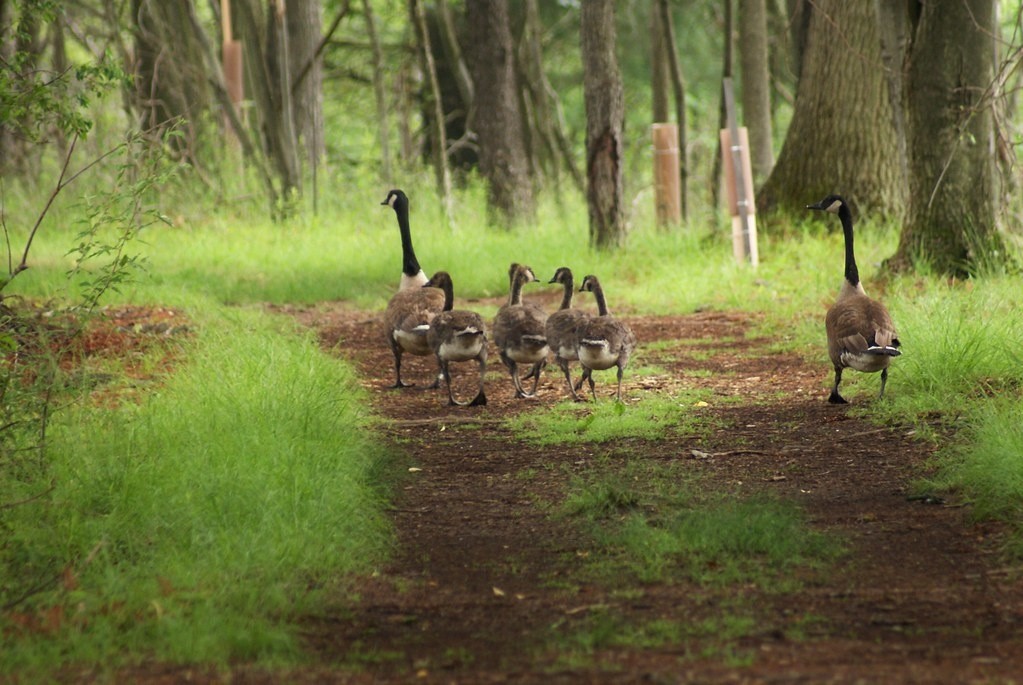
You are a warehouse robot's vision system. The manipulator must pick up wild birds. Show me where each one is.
[381,190,446,389]
[806,194,903,405]
[421,263,635,407]
